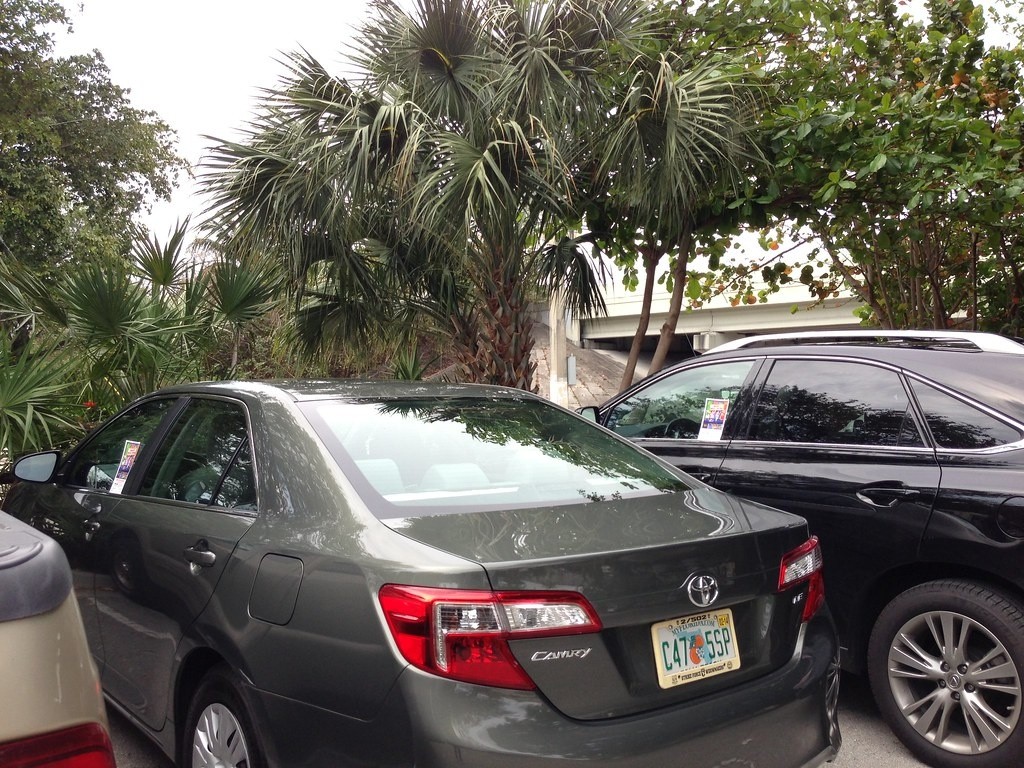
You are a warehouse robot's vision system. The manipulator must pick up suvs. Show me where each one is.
[570,331,1024,768]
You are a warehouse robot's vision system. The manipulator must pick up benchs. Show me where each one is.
[864,385,939,446]
[356,449,569,494]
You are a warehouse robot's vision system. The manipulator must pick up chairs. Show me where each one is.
[173,412,254,512]
[754,383,805,442]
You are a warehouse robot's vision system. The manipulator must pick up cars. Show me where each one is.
[1,379,844,768]
